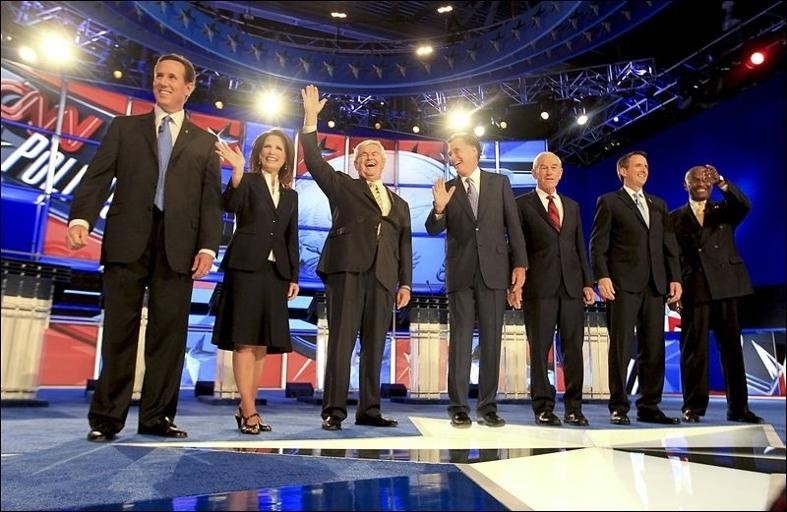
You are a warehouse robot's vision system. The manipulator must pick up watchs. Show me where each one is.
[716,174,726,188]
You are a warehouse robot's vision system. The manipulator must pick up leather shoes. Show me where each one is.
[532,410,561,427]
[477,412,507,428]
[87,429,116,442]
[449,411,473,430]
[319,415,342,431]
[609,411,631,426]
[636,409,702,425]
[355,416,399,427]
[136,425,189,438]
[564,413,590,426]
[725,402,768,424]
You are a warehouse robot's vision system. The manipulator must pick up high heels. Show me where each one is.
[232,400,271,436]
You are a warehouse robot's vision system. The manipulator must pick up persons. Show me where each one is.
[588,149,684,424]
[300,82,414,432]
[667,162,765,424]
[212,127,300,434]
[62,51,225,444]
[506,151,596,426]
[425,132,530,426]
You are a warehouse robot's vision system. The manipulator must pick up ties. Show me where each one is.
[149,114,179,214]
[694,201,706,227]
[464,179,481,227]
[365,178,385,243]
[633,193,650,230]
[545,195,561,234]
